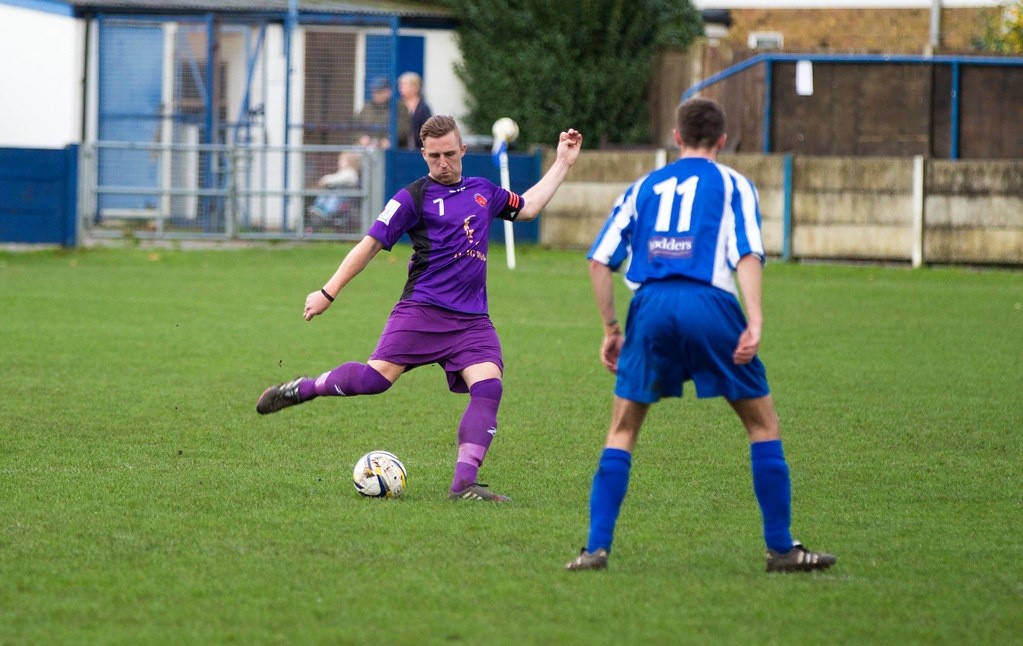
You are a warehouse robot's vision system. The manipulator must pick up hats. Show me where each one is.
[370,77,391,91]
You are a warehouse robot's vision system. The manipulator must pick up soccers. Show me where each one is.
[353,450,408,499]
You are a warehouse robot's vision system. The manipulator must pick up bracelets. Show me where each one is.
[320,289,336,302]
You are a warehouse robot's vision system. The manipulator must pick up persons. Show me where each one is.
[398,72,431,149]
[309,152,360,217]
[256,114,582,500]
[356,79,409,150]
[565,97,838,575]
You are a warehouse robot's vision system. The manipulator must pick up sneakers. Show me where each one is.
[564,546,608,572]
[256,375,309,414]
[447,482,511,501]
[765,543,836,573]
